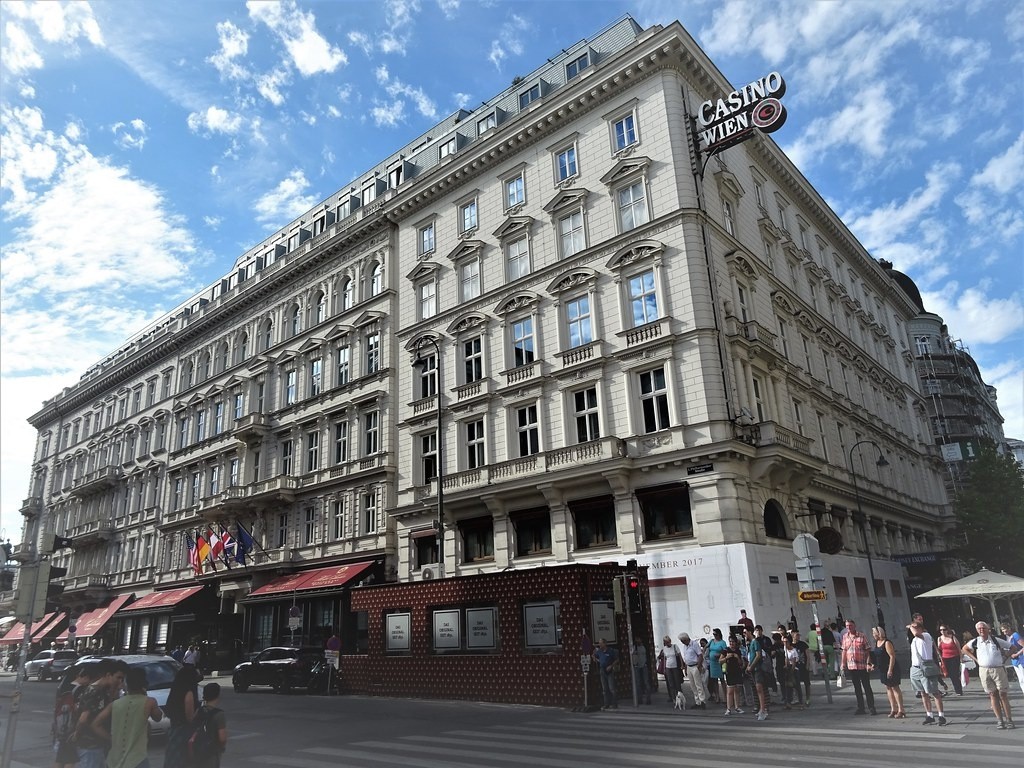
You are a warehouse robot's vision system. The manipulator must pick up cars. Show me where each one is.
[232,646,327,694]
[23,649,79,681]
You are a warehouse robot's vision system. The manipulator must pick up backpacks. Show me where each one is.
[184,702,223,762]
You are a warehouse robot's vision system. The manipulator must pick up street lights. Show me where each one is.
[849,440,892,639]
[416,337,446,579]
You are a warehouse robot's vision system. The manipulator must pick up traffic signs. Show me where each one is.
[798,589,826,602]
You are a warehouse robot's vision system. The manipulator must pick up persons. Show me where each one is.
[592,639,619,709]
[658,620,849,720]
[961,631,977,670]
[169,644,202,669]
[50,659,162,768]
[632,636,652,704]
[937,623,963,696]
[963,621,1017,729]
[906,613,944,698]
[999,622,1024,693]
[840,619,877,715]
[164,666,203,768]
[871,626,906,718]
[200,683,227,768]
[906,622,946,726]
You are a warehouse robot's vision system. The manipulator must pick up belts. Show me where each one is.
[688,664,696,667]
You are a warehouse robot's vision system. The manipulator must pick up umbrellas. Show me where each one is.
[914,566,1024,635]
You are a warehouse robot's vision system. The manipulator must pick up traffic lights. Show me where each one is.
[627,576,643,614]
[11,496,73,625]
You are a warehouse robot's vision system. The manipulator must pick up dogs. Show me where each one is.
[674,691,687,710]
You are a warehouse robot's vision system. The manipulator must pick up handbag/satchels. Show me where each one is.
[920,660,940,677]
[657,660,664,673]
[674,645,686,668]
[54,740,80,763]
[940,660,949,678]
[959,662,970,686]
[836,670,846,688]
[722,663,727,673]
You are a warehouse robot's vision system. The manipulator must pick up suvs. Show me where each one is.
[57,650,207,737]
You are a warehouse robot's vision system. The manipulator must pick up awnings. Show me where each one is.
[120,585,204,611]
[55,593,135,640]
[246,560,375,596]
[0,612,56,645]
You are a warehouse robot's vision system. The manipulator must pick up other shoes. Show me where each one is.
[609,704,617,709]
[870,707,876,715]
[755,710,768,720]
[1005,721,1015,729]
[997,721,1004,729]
[691,692,815,712]
[603,704,609,709]
[855,709,865,715]
[725,709,731,716]
[939,717,946,726]
[922,716,935,725]
[734,708,744,713]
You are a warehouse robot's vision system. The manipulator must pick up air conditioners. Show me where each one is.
[420,563,446,581]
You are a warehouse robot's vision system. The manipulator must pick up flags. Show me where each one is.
[221,528,236,562]
[235,525,253,564]
[186,534,199,571]
[207,528,224,560]
[196,531,210,562]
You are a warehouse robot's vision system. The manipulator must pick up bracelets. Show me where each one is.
[866,662,871,665]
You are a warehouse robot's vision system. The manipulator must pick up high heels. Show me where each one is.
[893,708,906,718]
[888,711,898,718]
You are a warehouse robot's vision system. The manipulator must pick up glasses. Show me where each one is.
[939,629,947,631]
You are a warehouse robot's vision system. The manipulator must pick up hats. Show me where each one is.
[203,683,220,700]
